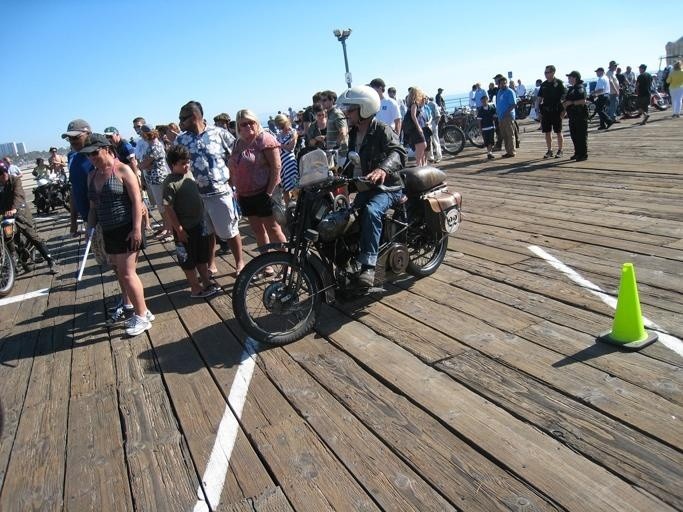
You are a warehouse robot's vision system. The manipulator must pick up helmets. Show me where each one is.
[0,160,8,174]
[338,86,380,119]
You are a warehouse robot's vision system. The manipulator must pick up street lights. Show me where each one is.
[334,27,353,88]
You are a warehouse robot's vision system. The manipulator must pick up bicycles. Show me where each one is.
[516,91,534,119]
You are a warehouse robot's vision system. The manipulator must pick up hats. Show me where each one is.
[567,71,581,78]
[80,133,111,153]
[103,127,118,135]
[141,124,156,132]
[595,68,604,72]
[610,61,619,65]
[62,120,91,139]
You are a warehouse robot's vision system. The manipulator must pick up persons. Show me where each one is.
[1,147,67,273]
[469,61,683,161]
[62,77,449,336]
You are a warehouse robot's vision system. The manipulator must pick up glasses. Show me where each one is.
[180,114,193,122]
[241,120,254,126]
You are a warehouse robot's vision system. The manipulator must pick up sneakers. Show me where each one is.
[571,154,587,161]
[544,150,554,158]
[487,153,494,159]
[358,265,375,287]
[598,119,619,129]
[502,154,515,158]
[555,150,563,158]
[107,298,154,336]
[48,261,59,273]
[640,115,649,124]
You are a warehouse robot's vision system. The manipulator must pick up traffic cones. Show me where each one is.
[596,263,661,354]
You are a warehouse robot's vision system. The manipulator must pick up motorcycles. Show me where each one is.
[436,105,487,155]
[0,204,39,296]
[586,73,671,122]
[231,146,462,347]
[32,167,72,214]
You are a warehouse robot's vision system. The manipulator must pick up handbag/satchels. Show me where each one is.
[403,110,420,133]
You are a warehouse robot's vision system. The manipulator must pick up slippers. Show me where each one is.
[190,282,221,298]
